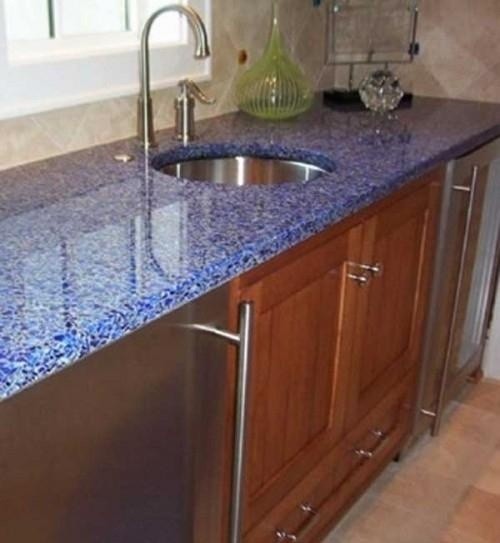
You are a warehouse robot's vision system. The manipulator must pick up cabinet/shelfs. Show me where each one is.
[224,164,447,540]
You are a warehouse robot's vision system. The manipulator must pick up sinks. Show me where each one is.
[147,137,338,189]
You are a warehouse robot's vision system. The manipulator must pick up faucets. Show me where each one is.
[134,2,210,147]
